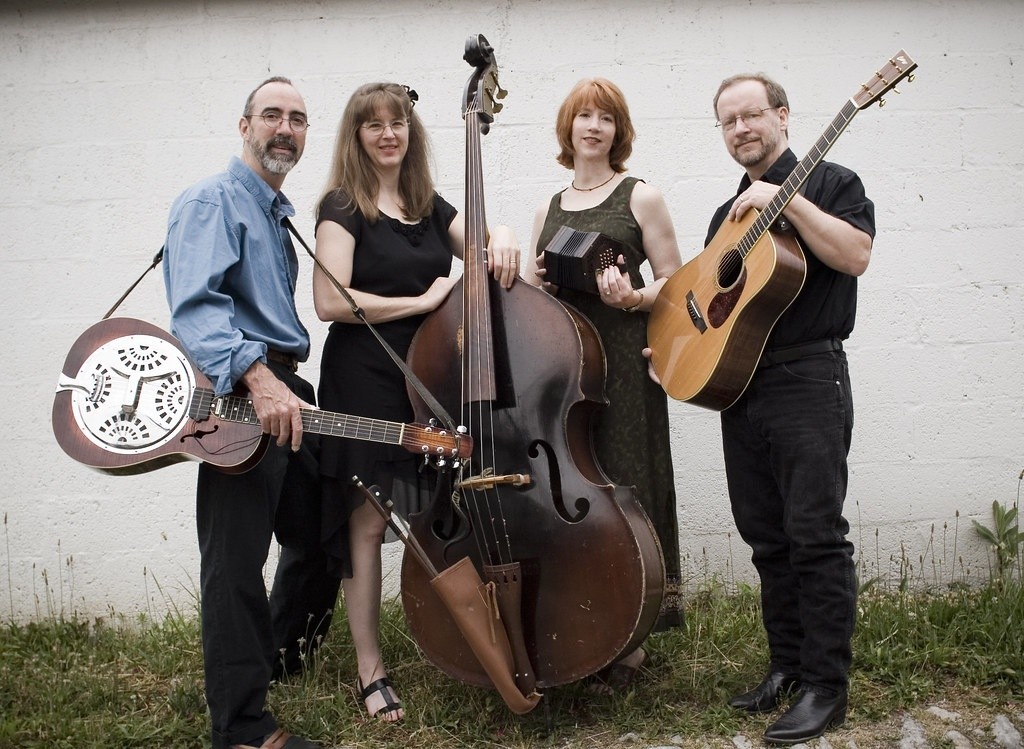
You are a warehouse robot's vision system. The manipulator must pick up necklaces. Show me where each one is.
[571,170,617,191]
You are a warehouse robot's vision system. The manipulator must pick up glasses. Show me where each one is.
[245,112,311,132]
[715,106,773,132]
[360,122,411,136]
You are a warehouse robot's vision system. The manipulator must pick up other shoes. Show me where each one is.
[226,728,322,749]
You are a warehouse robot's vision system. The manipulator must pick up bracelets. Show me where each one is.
[621,288,644,312]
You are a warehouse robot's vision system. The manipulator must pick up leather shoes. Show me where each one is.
[762,685,847,743]
[728,670,804,715]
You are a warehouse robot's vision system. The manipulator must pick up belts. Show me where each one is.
[263,350,298,372]
[757,339,843,366]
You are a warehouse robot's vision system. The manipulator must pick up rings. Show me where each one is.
[510,261,516,263]
[739,196,743,202]
[606,292,611,295]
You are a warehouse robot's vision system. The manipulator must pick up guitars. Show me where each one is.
[49,315,475,479]
[643,47,922,415]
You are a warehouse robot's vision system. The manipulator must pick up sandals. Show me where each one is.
[356,674,405,725]
[586,643,651,698]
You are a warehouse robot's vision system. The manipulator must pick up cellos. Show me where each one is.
[395,30,669,735]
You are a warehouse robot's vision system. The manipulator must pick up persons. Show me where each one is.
[522,80,683,698]
[646,72,876,742]
[313,82,519,722]
[163,77,332,749]
